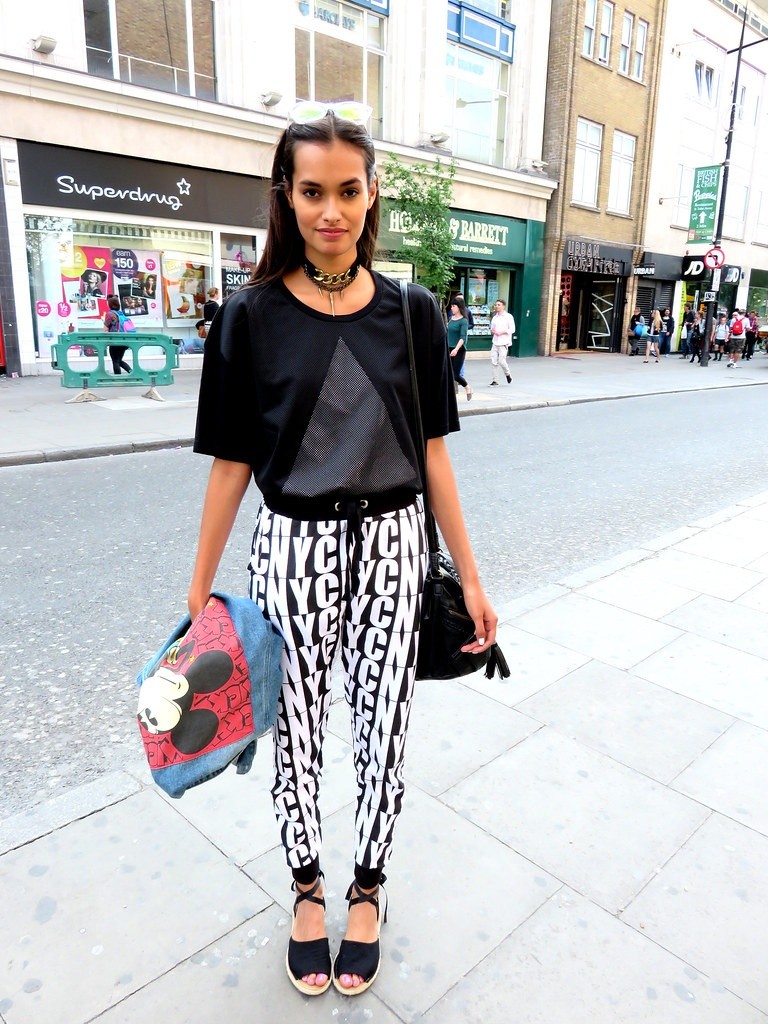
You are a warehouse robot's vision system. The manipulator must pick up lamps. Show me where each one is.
[260,90,283,108]
[525,156,549,173]
[427,132,451,147]
[31,34,58,53]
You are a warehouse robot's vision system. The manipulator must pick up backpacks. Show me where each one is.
[111,310,136,334]
[732,316,746,335]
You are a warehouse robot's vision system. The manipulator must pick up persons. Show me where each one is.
[628,307,645,356]
[487,299,515,388]
[81,270,107,297]
[680,303,758,369]
[447,298,472,402]
[652,307,674,357]
[643,309,663,363]
[103,295,132,375]
[188,101,497,996]
[204,287,219,336]
[143,275,155,298]
[449,293,474,376]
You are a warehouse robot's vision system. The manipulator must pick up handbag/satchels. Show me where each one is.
[634,323,649,338]
[691,325,703,349]
[414,551,510,684]
[681,324,687,338]
[198,325,206,338]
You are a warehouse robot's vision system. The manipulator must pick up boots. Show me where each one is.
[713,350,718,361]
[717,352,724,361]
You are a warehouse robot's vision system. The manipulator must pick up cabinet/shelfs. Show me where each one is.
[472,313,490,326]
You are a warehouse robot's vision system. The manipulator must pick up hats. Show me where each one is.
[739,309,746,313]
[82,268,108,283]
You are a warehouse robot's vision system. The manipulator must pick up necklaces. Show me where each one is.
[301,255,360,317]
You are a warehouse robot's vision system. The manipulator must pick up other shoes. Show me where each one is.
[507,374,511,383]
[467,387,473,401]
[629,347,711,364]
[490,382,499,388]
[727,361,734,367]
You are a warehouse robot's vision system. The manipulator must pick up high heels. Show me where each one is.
[286,874,335,996]
[330,881,391,994]
